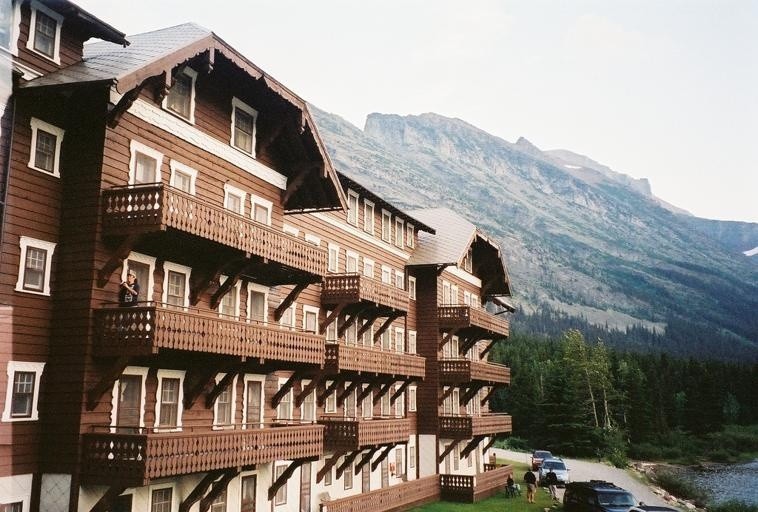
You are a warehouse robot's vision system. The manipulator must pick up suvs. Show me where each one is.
[627,504,680,512]
[561,477,641,512]
[530,449,555,470]
[538,456,569,486]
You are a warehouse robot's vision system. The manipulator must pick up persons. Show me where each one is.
[524,466,538,504]
[507,473,521,496]
[545,468,559,500]
[119,269,139,338]
[490,452,497,470]
